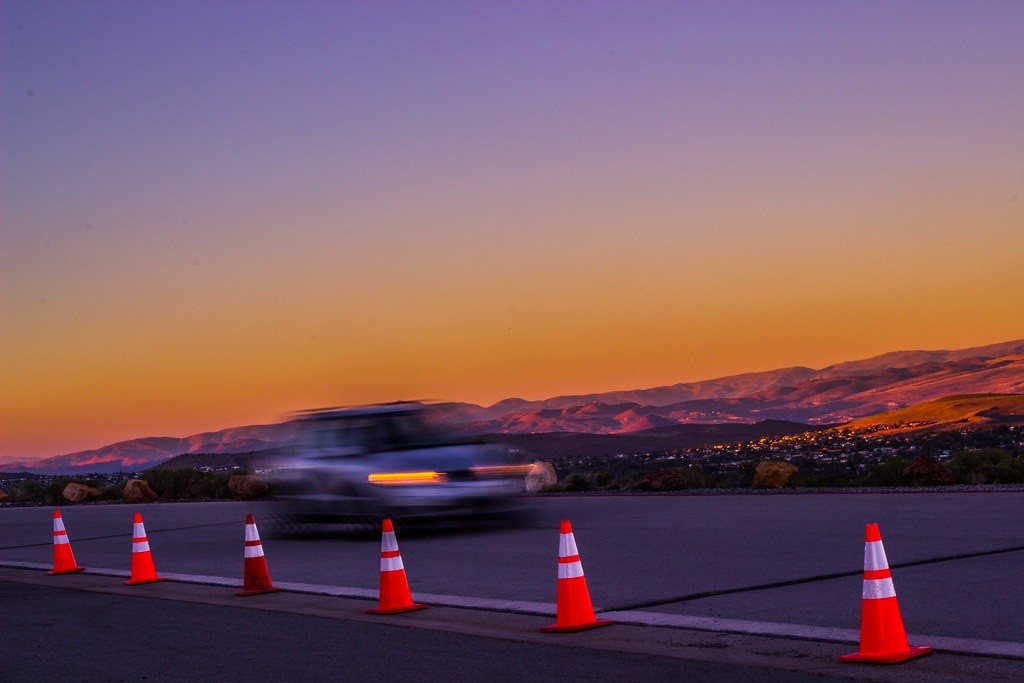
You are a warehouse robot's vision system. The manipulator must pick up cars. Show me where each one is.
[278,401,559,533]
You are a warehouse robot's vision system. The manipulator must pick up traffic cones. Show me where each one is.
[361,517,427,615]
[121,511,168,586]
[838,523,930,662]
[231,514,286,597]
[539,519,612,630]
[45,510,88,574]
